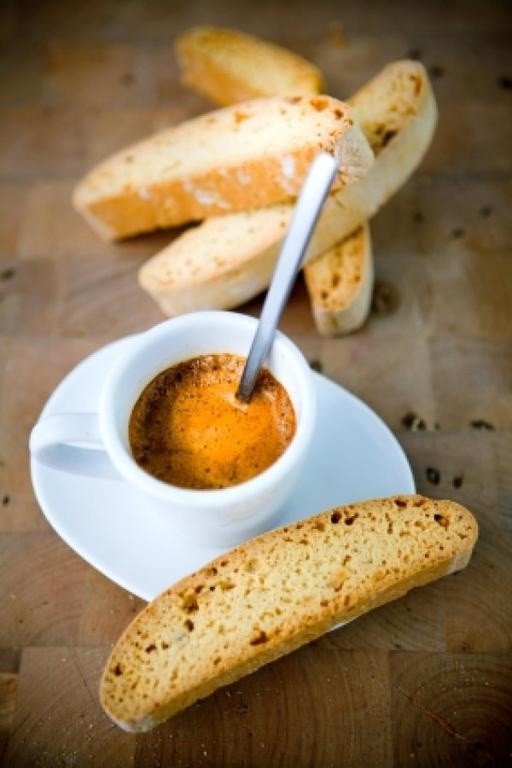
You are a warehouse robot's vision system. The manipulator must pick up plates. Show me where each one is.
[28,334,416,634]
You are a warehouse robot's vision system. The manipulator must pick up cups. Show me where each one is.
[31,308,320,548]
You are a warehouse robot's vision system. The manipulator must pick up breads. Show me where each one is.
[72,90,375,245]
[301,223,374,336]
[137,60,439,315]
[177,27,323,107]
[98,494,479,734]
